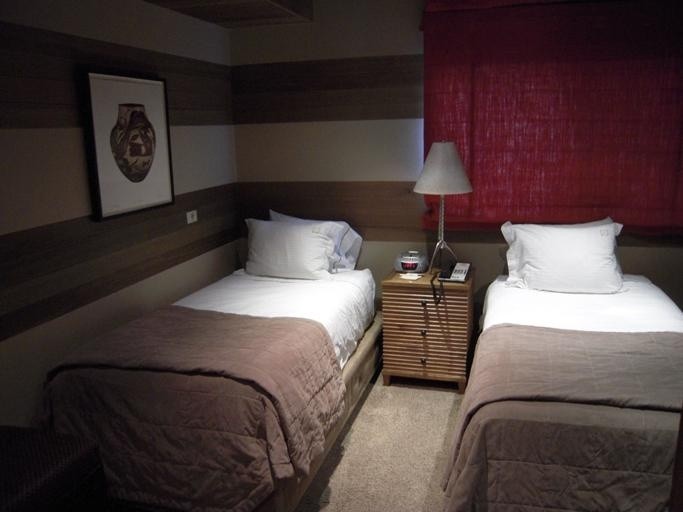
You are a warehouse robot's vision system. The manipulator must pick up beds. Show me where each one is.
[30,266,387,511]
[472,272,683,509]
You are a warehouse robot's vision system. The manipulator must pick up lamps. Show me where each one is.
[410,140,472,277]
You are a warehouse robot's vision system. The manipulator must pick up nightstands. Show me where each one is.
[377,264,476,398]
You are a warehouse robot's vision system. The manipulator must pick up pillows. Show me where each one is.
[500,220,627,296]
[556,213,612,228]
[266,208,362,276]
[240,216,351,285]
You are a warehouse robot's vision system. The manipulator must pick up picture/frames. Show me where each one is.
[83,67,177,223]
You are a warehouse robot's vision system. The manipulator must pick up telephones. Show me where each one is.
[438,259,472,282]
